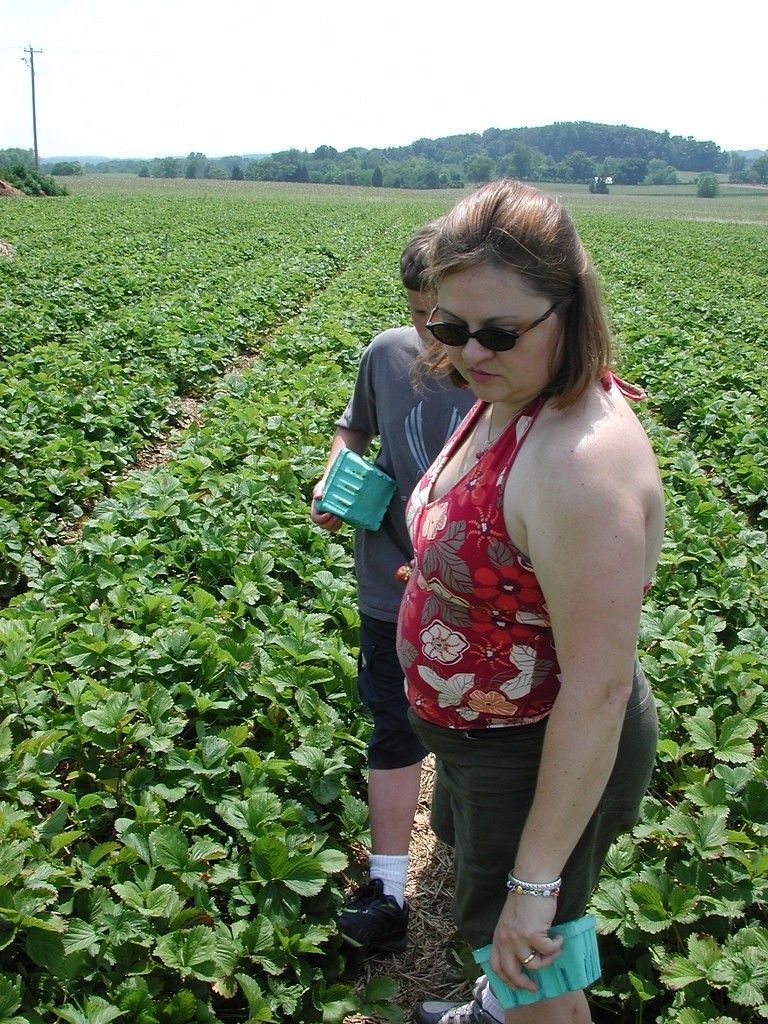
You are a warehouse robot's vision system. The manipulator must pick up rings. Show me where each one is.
[519,949,536,964]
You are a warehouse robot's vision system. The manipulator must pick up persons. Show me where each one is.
[395,181,664,1023]
[310,219,475,953]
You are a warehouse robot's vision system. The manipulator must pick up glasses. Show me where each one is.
[425,303,561,354]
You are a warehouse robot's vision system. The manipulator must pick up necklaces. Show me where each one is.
[475,399,530,462]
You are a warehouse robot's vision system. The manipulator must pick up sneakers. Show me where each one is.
[415,974,502,1022]
[334,877,412,953]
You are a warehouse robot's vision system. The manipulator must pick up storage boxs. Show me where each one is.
[314,447,398,532]
[471,914,603,1008]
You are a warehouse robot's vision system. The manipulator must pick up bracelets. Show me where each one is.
[506,871,562,898]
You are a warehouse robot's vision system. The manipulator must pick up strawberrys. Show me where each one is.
[395,564,411,582]
